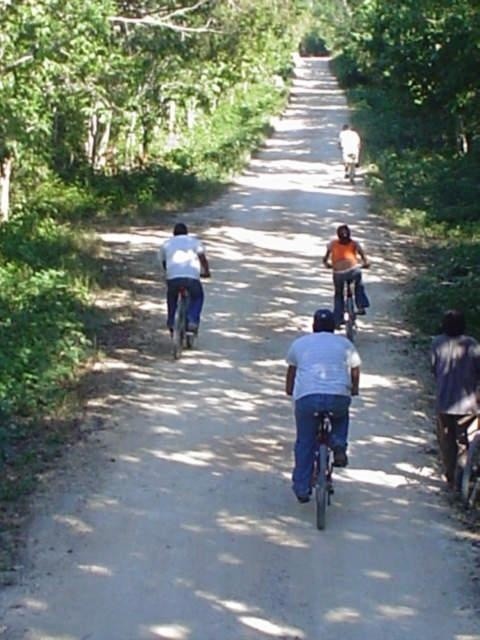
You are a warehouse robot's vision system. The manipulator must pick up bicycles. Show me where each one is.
[336,141,357,183]
[287,389,356,528]
[318,262,371,342]
[164,269,210,361]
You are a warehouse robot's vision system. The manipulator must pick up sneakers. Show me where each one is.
[334,447,346,466]
[360,306,364,312]
[297,486,312,503]
[187,325,196,333]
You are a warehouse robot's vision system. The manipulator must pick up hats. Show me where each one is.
[442,309,464,330]
[314,310,331,322]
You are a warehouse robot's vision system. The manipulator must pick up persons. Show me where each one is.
[321,225,371,328]
[427,309,480,494]
[337,124,361,179]
[157,222,211,339]
[285,309,364,503]
